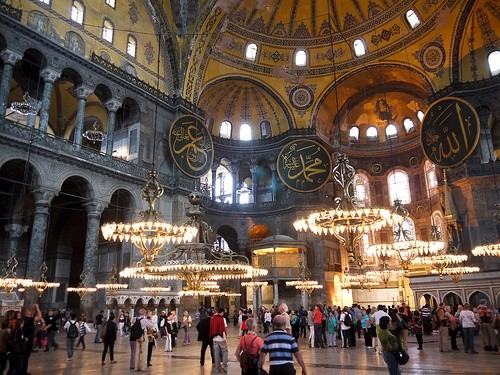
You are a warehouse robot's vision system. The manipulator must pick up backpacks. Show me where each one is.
[239,336,258,371]
[130,317,145,339]
[343,312,352,326]
[67,321,79,339]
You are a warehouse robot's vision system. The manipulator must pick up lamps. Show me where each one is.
[10,62,39,116]
[0,182,61,299]
[286,0,500,299]
[67,0,269,300]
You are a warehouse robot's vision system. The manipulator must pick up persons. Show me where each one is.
[101,314,117,365]
[378,316,401,375]
[0,299,500,375]
[209,308,229,373]
[235,318,265,375]
[257,315,307,375]
[63,313,79,361]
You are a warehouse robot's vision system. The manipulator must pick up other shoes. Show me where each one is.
[101,362,105,365]
[222,365,227,373]
[417,347,423,350]
[54,344,58,351]
[43,349,49,352]
[110,361,117,365]
[216,369,222,373]
[452,347,459,351]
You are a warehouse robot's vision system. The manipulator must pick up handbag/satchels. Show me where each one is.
[391,348,410,365]
[12,343,23,355]
[100,324,107,339]
